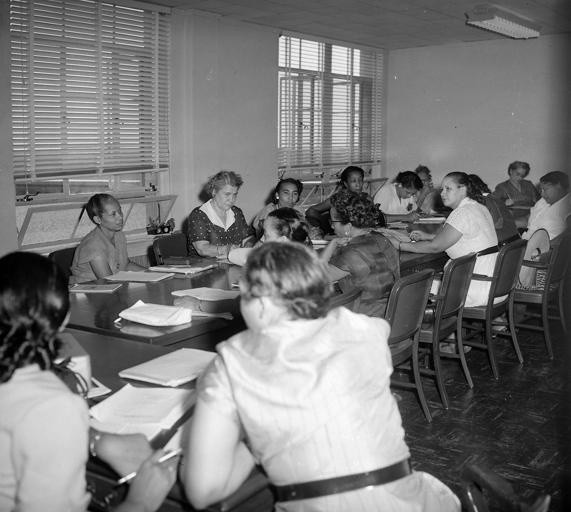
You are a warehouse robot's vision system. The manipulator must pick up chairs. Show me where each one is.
[330,285,362,316]
[388,268,435,424]
[47,246,77,268]
[152,234,188,267]
[418,251,478,411]
[515,233,570,361]
[463,239,526,381]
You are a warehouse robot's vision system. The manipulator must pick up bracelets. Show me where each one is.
[399,241,402,252]
[89,432,102,457]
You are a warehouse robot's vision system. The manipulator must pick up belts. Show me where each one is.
[273,459,411,503]
[499,234,519,246]
[477,245,499,257]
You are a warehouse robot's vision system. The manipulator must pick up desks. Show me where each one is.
[54,324,272,512]
[66,217,446,347]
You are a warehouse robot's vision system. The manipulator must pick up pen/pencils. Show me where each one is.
[506,192,511,199]
[117,447,181,485]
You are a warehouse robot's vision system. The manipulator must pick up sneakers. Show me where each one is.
[440,342,456,355]
[492,318,507,332]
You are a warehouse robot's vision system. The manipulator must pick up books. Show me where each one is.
[118,347,218,389]
[413,216,446,224]
[69,284,123,293]
[104,271,175,282]
[87,384,196,442]
[171,286,240,320]
[149,264,219,274]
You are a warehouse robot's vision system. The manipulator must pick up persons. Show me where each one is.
[319,189,401,318]
[386,172,509,354]
[186,171,256,259]
[373,171,426,223]
[0,251,180,512]
[473,174,521,334]
[494,161,540,206]
[180,240,463,512]
[69,194,147,286]
[259,206,315,250]
[521,172,570,240]
[414,164,443,216]
[306,166,375,235]
[252,178,307,238]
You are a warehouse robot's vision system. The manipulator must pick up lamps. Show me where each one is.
[464,7,542,39]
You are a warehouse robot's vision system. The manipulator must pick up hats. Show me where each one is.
[518,228,550,288]
